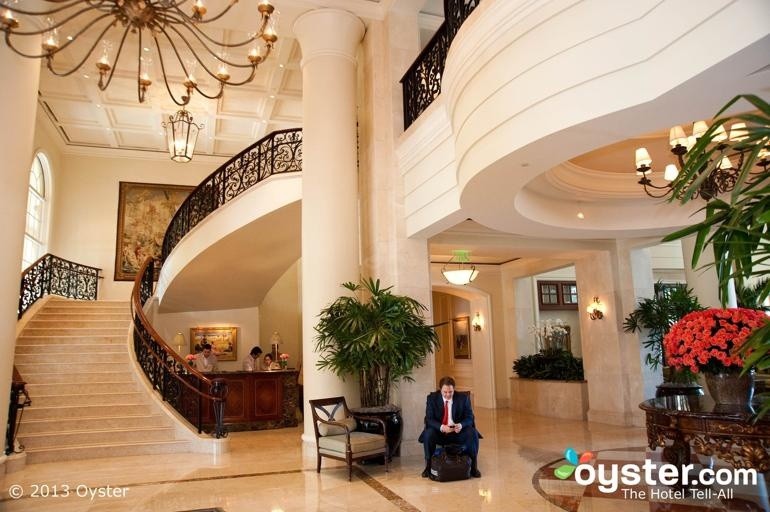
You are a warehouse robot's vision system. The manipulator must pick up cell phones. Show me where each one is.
[450,425,456,428]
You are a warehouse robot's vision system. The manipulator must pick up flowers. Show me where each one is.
[277,352,289,367]
[183,353,197,366]
[657,303,770,377]
[528,318,571,354]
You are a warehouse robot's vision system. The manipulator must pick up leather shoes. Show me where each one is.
[422,466,431,477]
[472,469,481,478]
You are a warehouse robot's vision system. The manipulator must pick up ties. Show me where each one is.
[442,401,448,426]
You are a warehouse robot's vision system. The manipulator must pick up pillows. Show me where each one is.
[318,418,359,436]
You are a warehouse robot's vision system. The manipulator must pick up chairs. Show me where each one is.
[308,394,391,482]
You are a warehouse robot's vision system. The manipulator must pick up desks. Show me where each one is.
[638,392,770,496]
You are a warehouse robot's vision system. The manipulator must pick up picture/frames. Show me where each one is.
[451,314,473,361]
[188,325,240,362]
[111,179,220,284]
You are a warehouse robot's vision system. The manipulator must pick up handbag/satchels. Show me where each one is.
[431,446,471,481]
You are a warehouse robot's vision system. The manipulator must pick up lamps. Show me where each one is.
[472,309,484,335]
[171,330,187,356]
[162,96,206,165]
[268,329,286,370]
[588,295,607,322]
[437,250,481,285]
[1,0,283,107]
[625,119,770,202]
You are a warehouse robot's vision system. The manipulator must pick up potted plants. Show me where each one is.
[621,276,710,398]
[312,275,442,465]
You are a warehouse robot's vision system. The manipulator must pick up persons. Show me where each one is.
[270,343,282,362]
[242,346,263,371]
[261,353,276,370]
[193,344,217,371]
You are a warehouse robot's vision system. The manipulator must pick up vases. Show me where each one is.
[699,366,754,415]
